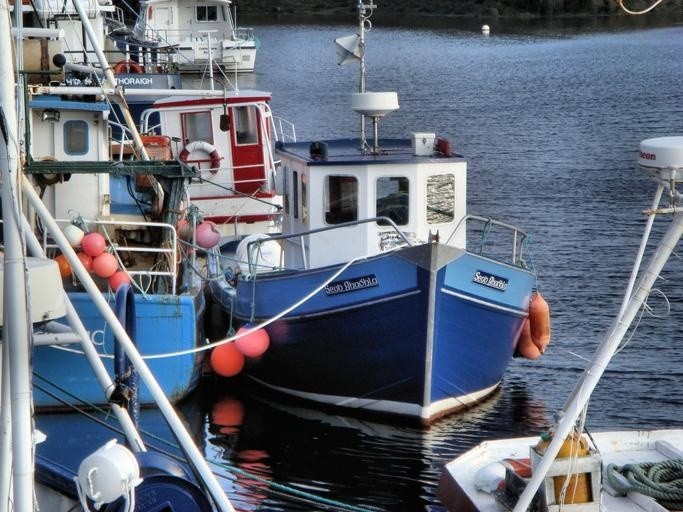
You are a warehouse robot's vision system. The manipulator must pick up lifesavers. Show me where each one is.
[181,142,220,183]
[114,59,144,74]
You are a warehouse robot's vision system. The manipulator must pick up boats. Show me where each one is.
[205,2,537,432]
[0,0,298,512]
[435,123,683,512]
